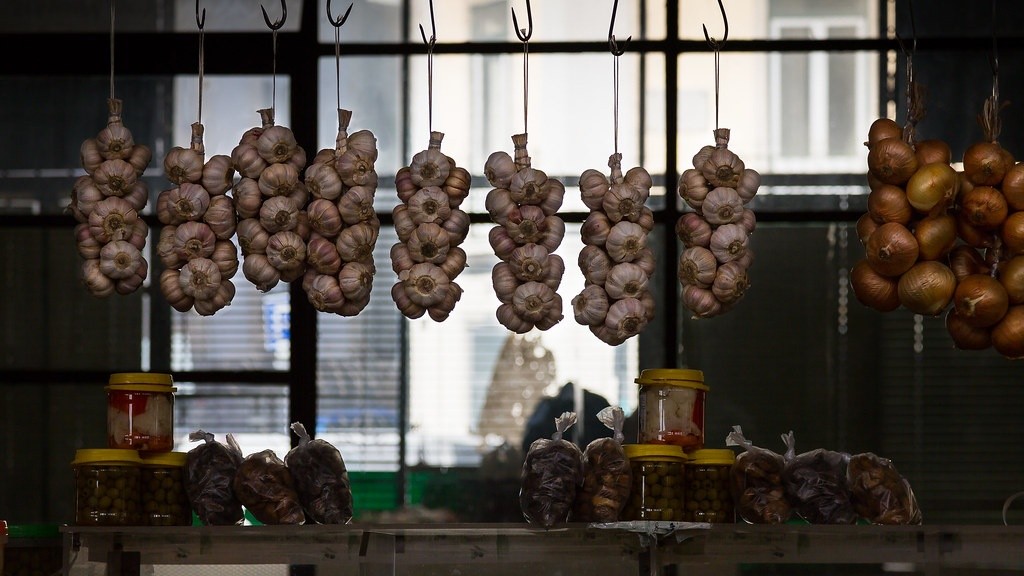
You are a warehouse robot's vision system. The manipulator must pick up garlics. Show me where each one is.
[75,100,759,345]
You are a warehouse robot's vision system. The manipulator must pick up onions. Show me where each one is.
[851,118,1024,358]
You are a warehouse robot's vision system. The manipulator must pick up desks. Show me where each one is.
[59,523,1024,576]
